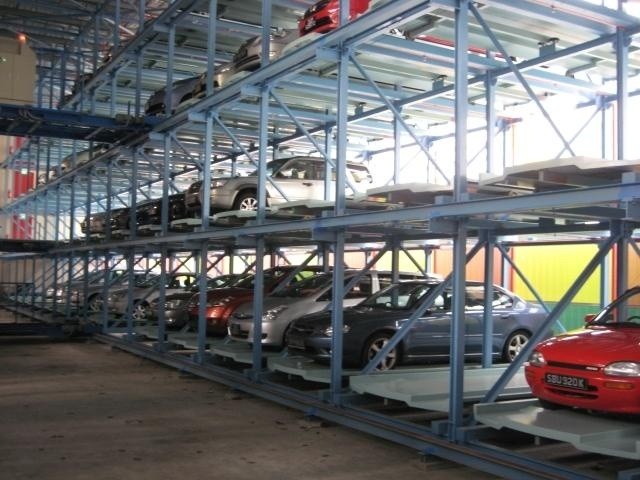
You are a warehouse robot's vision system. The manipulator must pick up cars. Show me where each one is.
[519,283,640,422]
